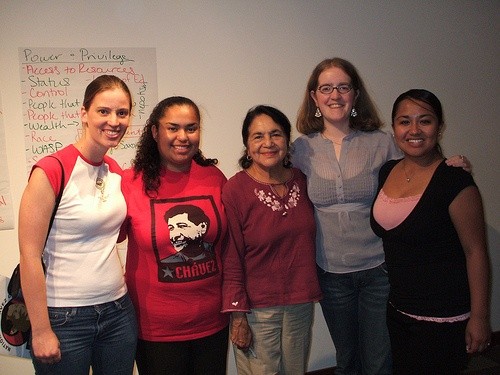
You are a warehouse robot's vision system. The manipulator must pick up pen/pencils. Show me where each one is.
[248,348,256,357]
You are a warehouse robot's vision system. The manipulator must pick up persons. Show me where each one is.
[19,75,139,375]
[289,58,473,375]
[220,105,323,375]
[369,89,492,375]
[116,97,230,375]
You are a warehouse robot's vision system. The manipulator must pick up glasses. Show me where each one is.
[315,84,355,95]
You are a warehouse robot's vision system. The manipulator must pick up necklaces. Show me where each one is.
[403,159,434,181]
[322,133,343,146]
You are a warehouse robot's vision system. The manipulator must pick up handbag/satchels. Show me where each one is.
[6,258,47,305]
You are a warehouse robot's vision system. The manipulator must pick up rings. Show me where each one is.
[487,342,490,345]
